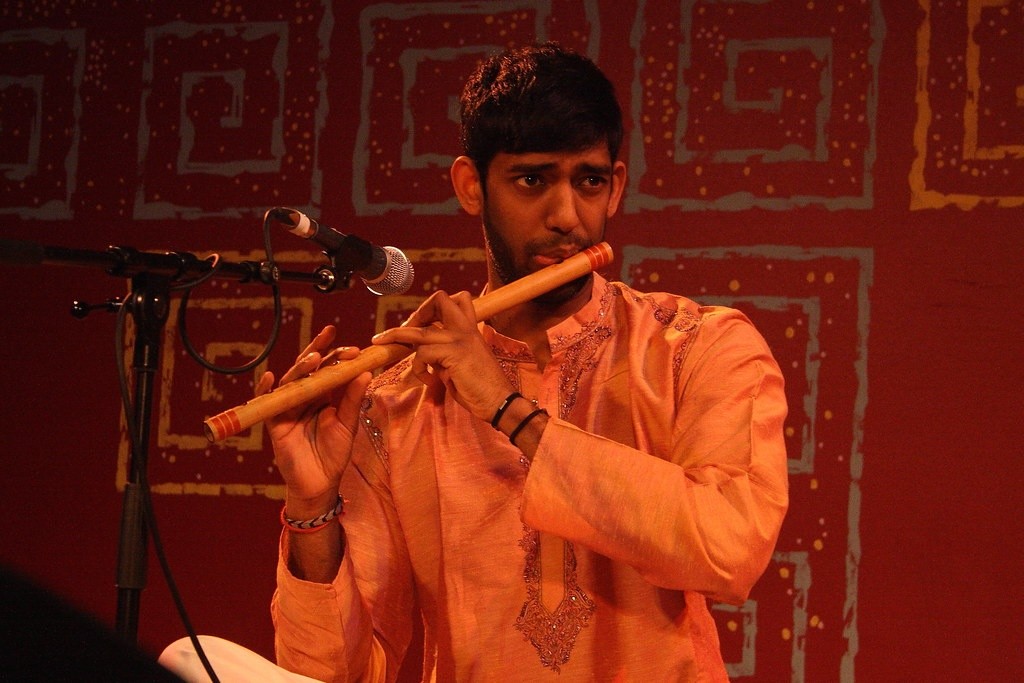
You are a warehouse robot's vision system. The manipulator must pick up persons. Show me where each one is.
[257,39,789,683]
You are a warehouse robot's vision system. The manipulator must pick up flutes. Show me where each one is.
[204,240,612,442]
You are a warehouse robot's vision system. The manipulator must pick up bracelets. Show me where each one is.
[508,409,549,446]
[278,496,355,535]
[489,391,522,429]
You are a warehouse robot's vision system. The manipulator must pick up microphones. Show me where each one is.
[273,206,415,298]
